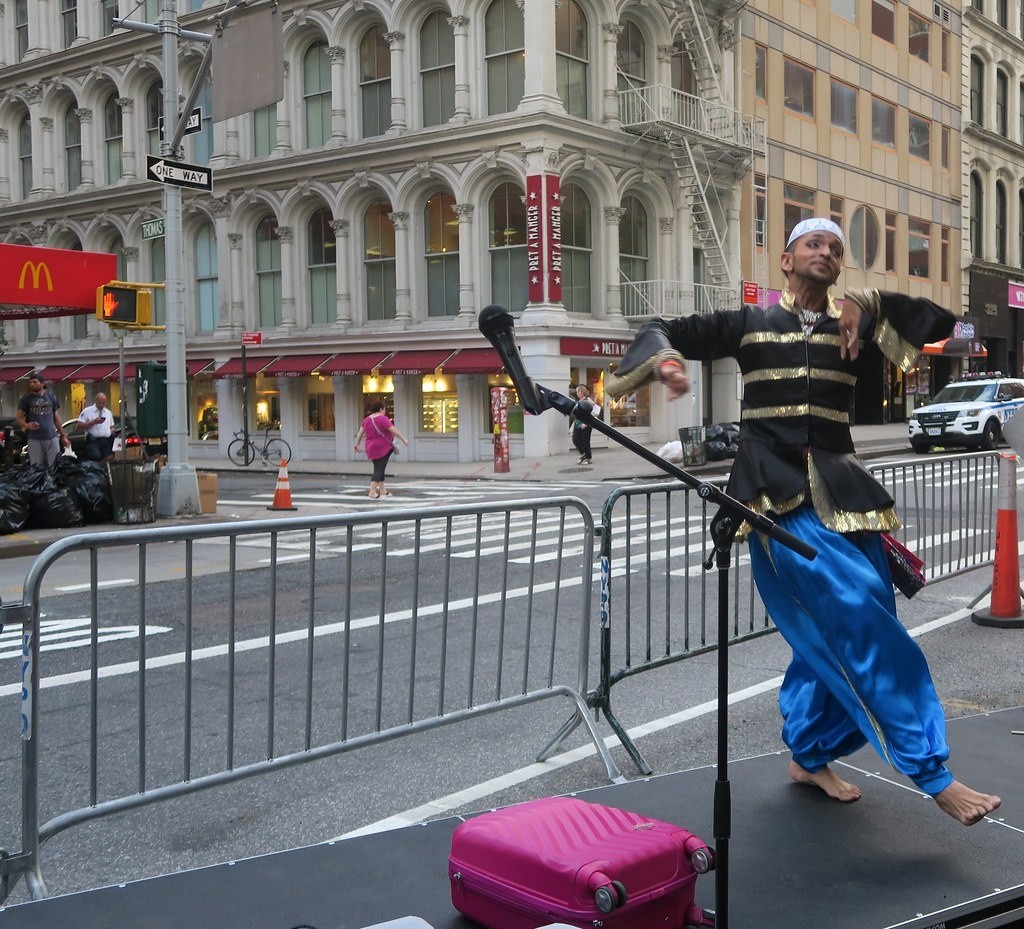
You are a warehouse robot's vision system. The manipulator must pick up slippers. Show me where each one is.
[367,495,383,501]
[379,491,392,496]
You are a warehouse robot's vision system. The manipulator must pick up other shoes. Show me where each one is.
[577,453,592,465]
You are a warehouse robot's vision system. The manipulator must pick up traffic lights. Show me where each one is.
[139,291,152,325]
[95,284,140,325]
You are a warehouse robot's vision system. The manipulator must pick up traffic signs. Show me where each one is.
[157,108,202,141]
[145,157,212,194]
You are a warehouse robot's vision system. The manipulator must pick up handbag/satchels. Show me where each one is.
[392,437,400,455]
[591,404,601,417]
[568,422,574,438]
[112,435,128,453]
[62,445,77,459]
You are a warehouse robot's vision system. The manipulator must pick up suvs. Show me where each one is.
[909,371,1024,454]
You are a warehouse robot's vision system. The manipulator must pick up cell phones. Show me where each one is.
[101,417,107,420]
[33,422,39,428]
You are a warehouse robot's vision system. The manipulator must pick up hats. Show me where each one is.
[784,218,845,261]
[30,374,47,389]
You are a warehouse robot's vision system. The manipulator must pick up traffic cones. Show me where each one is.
[266,458,298,511]
[971,453,1023,629]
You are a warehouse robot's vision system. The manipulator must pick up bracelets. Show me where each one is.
[26,423,29,430]
[60,433,67,437]
[657,359,686,380]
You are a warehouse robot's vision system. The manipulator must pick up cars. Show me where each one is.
[0,416,27,464]
[21,418,166,462]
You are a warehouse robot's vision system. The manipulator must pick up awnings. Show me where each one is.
[211,348,506,380]
[920,335,987,358]
[0,358,215,382]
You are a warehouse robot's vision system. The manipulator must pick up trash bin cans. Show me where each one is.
[108,460,159,524]
[679,427,708,467]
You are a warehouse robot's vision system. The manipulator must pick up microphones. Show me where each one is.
[478,304,544,415]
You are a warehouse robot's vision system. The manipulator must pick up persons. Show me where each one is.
[75,392,116,462]
[15,374,72,470]
[354,401,408,498]
[571,385,595,466]
[602,213,1005,827]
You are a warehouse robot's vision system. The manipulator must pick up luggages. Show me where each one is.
[448,797,716,929]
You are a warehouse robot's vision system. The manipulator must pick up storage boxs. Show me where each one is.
[195,471,217,512]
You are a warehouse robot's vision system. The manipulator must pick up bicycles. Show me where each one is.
[227,426,292,466]
